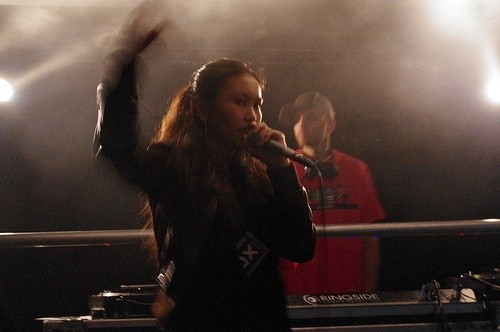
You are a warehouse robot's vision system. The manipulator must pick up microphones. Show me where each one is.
[245,127,317,168]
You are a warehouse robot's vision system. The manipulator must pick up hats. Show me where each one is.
[279,92,334,127]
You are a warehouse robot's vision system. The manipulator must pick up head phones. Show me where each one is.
[305,151,338,178]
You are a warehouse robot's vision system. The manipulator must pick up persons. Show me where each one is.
[92,7,318,332]
[272,91,387,297]
